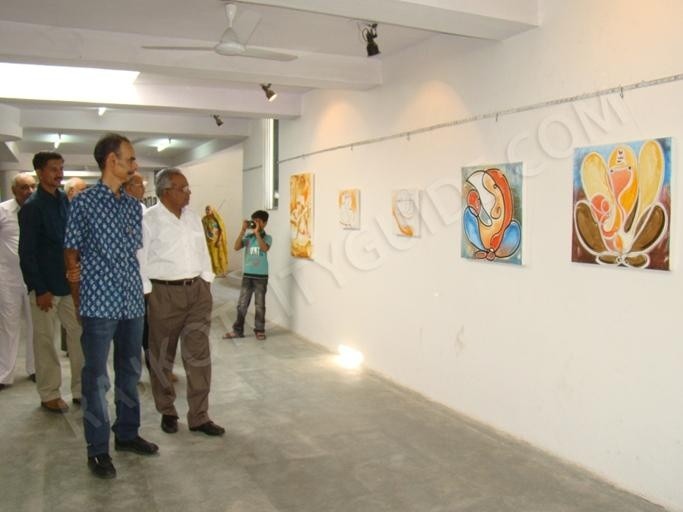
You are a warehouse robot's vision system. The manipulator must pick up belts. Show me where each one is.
[150,276,201,288]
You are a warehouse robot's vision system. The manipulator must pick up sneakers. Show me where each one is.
[88,453,116,479]
[114,434,161,455]
[0,350,82,405]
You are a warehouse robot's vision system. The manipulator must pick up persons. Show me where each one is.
[123,172,178,382]
[64,177,88,201]
[18,152,84,413]
[201,205,228,276]
[0,173,39,389]
[221,211,272,340]
[65,134,158,477]
[137,168,226,436]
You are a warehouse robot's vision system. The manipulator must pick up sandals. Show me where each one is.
[222,331,245,339]
[255,330,266,341]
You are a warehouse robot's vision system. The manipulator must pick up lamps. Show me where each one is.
[362,23,381,57]
[213,114,225,129]
[260,83,277,102]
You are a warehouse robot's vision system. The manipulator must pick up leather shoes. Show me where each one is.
[189,420,226,437]
[41,398,69,413]
[160,413,179,434]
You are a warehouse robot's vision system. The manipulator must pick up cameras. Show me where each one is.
[246,221,255,228]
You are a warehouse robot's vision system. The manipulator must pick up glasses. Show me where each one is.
[164,185,189,194]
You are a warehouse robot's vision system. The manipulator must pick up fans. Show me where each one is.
[141,4,299,62]
